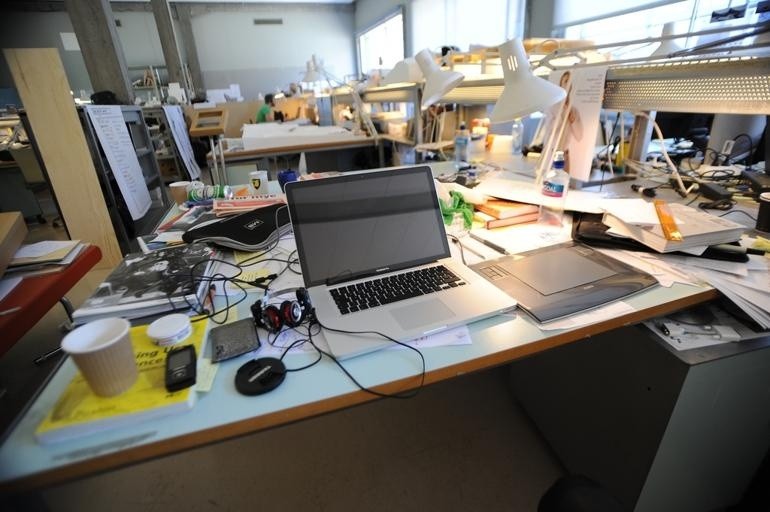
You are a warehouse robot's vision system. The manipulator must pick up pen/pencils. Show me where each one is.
[202,284,216,315]
[468,231,510,255]
[34,347,62,364]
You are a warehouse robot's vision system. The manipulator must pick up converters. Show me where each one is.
[699,182,733,203]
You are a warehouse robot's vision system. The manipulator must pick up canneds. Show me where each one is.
[188,185,233,201]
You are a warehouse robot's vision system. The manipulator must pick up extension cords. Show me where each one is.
[697,164,741,177]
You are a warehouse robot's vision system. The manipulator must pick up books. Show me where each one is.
[34,314,210,447]
[71,241,223,328]
[602,203,747,254]
[474,200,539,229]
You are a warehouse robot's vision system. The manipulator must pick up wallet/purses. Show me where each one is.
[209,317,261,364]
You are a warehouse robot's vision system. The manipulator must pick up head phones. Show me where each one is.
[251,286,308,333]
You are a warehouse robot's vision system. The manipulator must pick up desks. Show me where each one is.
[0,211,103,370]
[203,127,388,181]
[0,163,770,512]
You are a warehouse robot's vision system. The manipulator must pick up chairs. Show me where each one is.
[0,151,48,229]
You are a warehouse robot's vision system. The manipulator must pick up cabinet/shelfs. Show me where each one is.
[128,64,173,90]
[76,105,170,255]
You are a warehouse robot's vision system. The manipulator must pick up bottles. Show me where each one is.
[454,122,470,171]
[536,152,569,240]
[512,117,524,154]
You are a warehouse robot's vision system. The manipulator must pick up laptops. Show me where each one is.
[283,164,517,360]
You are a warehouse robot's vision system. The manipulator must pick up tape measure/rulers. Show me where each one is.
[653,199,683,241]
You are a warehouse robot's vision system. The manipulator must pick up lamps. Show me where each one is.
[301,54,364,96]
[486,35,582,127]
[415,49,468,114]
[273,84,304,99]
[648,21,688,56]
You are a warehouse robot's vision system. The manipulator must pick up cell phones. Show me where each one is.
[165,344,195,392]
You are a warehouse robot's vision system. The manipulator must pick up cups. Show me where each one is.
[754,192,770,234]
[276,170,297,192]
[249,170,269,196]
[169,181,190,207]
[61,317,139,400]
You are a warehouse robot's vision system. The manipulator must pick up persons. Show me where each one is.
[255,94,277,123]
[544,72,583,173]
